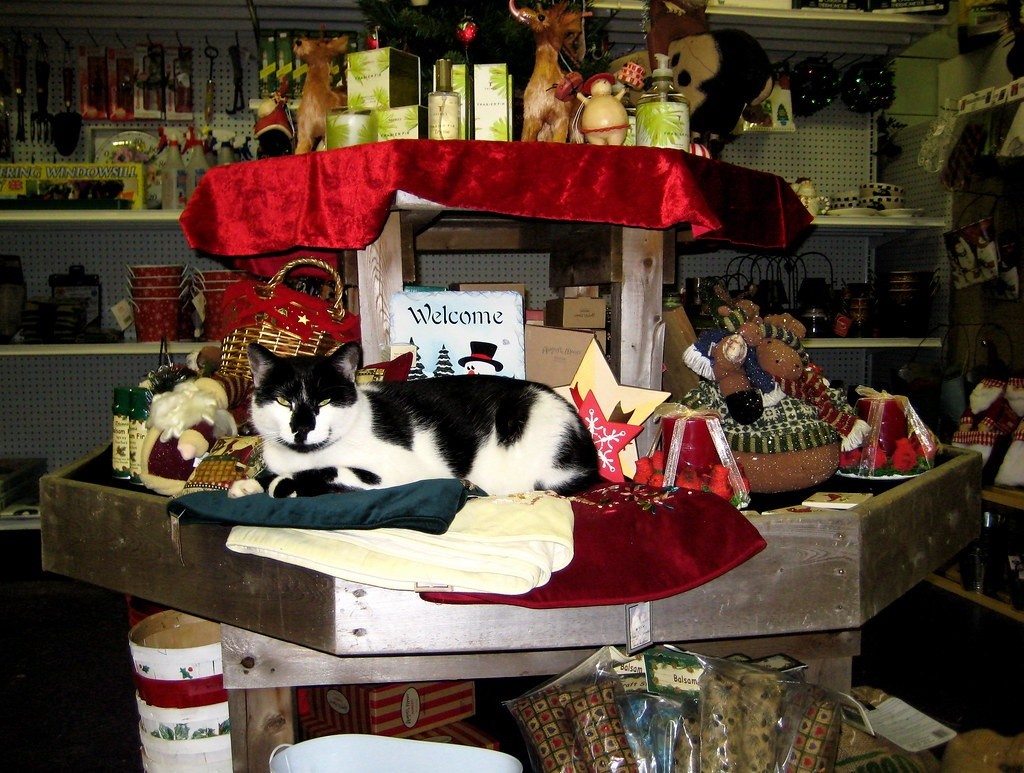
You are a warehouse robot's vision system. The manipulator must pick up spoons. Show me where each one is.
[53,38,82,157]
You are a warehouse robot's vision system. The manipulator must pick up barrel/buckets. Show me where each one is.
[128,609,235,773]
[265,734,523,773]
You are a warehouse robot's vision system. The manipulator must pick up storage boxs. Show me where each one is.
[123,260,248,344]
[296,680,500,756]
[326,47,515,145]
[451,278,608,392]
[216,260,363,383]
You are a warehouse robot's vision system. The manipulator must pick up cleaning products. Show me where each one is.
[635,50,691,153]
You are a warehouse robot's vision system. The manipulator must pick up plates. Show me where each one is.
[95,131,162,207]
[827,208,877,217]
[877,209,925,217]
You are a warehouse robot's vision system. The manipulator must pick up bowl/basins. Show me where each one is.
[830,201,860,209]
[861,183,906,197]
[859,195,906,208]
[831,190,859,201]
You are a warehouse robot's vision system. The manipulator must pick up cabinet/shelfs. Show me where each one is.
[38,138,982,773]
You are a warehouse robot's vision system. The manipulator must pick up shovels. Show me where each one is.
[53,68,82,157]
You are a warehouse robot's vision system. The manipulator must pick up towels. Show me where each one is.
[224,493,572,594]
[163,478,487,539]
[420,480,770,611]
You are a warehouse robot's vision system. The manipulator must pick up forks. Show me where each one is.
[30,42,53,147]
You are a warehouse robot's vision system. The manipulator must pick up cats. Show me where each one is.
[227,343,600,498]
